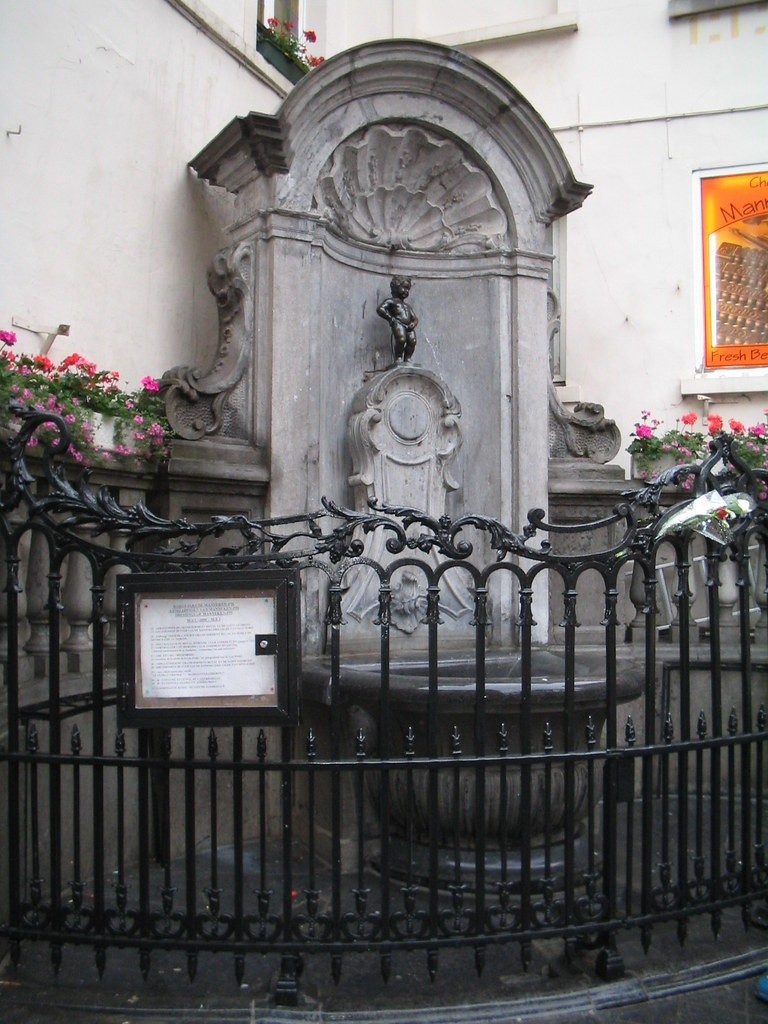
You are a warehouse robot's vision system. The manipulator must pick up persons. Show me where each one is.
[376,275,421,367]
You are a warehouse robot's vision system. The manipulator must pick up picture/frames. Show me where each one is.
[693,163,768,376]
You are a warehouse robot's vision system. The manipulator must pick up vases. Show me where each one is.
[0,410,168,472]
[629,451,709,481]
[255,39,309,85]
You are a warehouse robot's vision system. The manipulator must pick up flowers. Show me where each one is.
[625,409,768,500]
[0,329,176,465]
[266,17,329,67]
[614,490,759,558]
